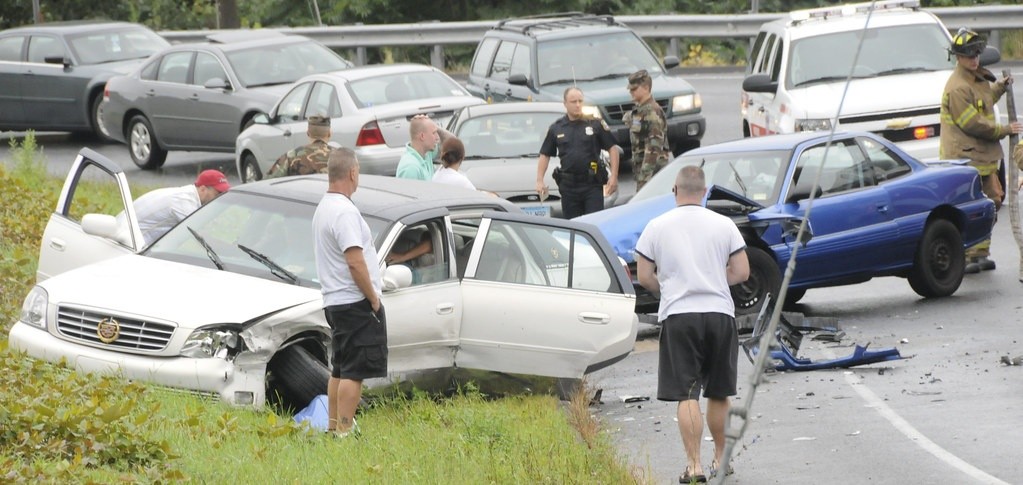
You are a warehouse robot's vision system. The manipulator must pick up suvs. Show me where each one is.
[468,16,707,154]
[741,0,1006,206]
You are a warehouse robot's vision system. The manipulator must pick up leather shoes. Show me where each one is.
[963,257,996,275]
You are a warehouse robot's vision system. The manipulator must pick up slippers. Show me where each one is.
[678,465,707,483]
[710,463,735,476]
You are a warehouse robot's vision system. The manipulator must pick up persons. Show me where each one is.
[384,230,432,265]
[396,115,477,190]
[114,169,231,250]
[536,87,620,220]
[635,167,750,483]
[312,148,389,445]
[622,69,675,191]
[265,114,336,178]
[940,28,1023,274]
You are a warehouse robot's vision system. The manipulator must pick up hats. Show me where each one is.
[440,136,465,159]
[307,112,331,126]
[194,169,230,192]
[626,70,648,89]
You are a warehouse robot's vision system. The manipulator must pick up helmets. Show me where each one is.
[948,28,987,56]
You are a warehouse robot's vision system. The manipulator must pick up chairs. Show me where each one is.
[385,82,410,102]
[165,64,188,82]
[794,166,828,194]
[392,238,421,285]
[467,133,499,156]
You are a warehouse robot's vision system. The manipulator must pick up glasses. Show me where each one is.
[952,41,986,57]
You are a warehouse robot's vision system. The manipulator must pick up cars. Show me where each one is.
[0,20,176,143]
[235,62,489,184]
[554,127,997,321]
[446,104,616,220]
[9,146,639,409]
[102,31,358,168]
